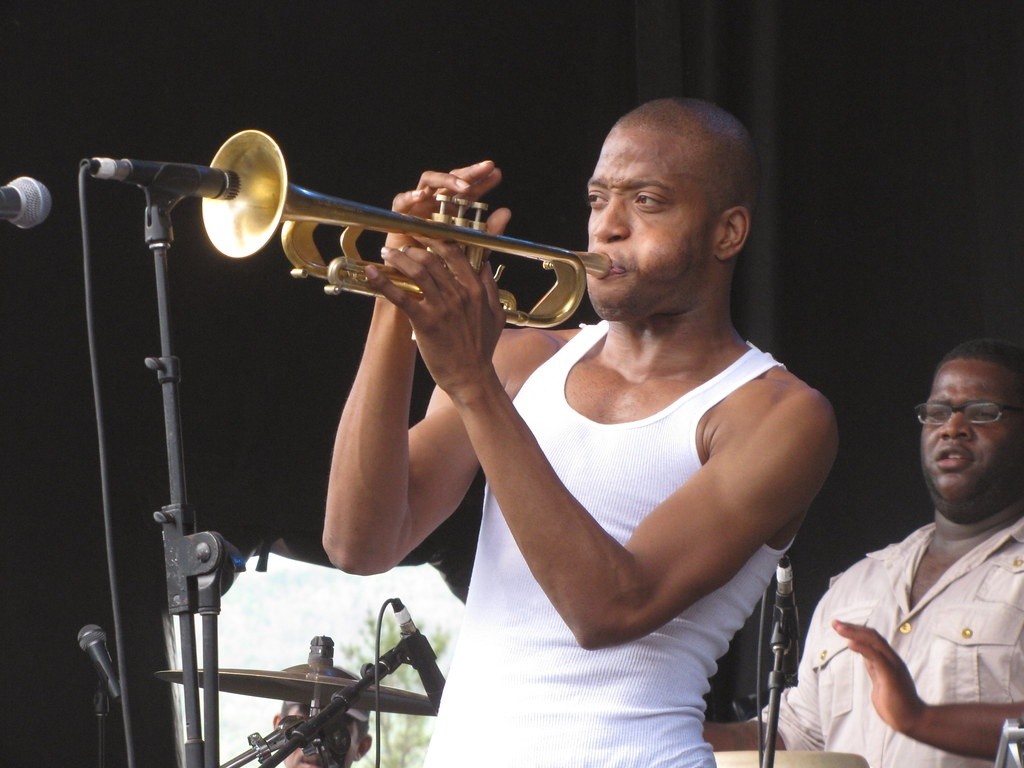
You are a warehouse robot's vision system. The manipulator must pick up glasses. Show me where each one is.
[912,402,1024,425]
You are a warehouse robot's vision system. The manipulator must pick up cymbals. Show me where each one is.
[151,664,439,717]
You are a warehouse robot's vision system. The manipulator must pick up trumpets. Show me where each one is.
[200,130,612,333]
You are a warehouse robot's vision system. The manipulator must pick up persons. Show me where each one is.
[322,100,838,768]
[704,339,1024,768]
[273,698,372,768]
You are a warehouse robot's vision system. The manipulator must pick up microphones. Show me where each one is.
[0,174,54,232]
[389,596,446,716]
[775,554,802,688]
[74,621,122,703]
[82,154,243,201]
